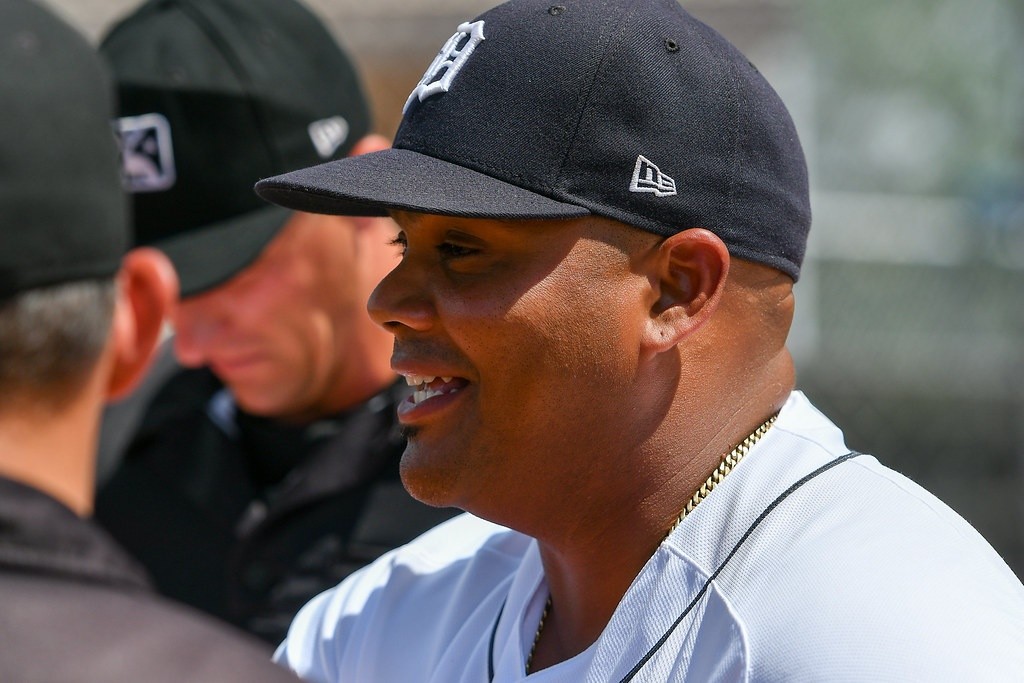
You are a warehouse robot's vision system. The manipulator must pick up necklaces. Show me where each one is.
[524,415,778,671]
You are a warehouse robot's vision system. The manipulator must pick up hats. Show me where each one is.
[0,0,127,296]
[96,0,372,299]
[254,0,812,282]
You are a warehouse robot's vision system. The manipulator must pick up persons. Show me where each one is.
[255,0,1024,683]
[0,0,302,683]
[92,0,467,664]
